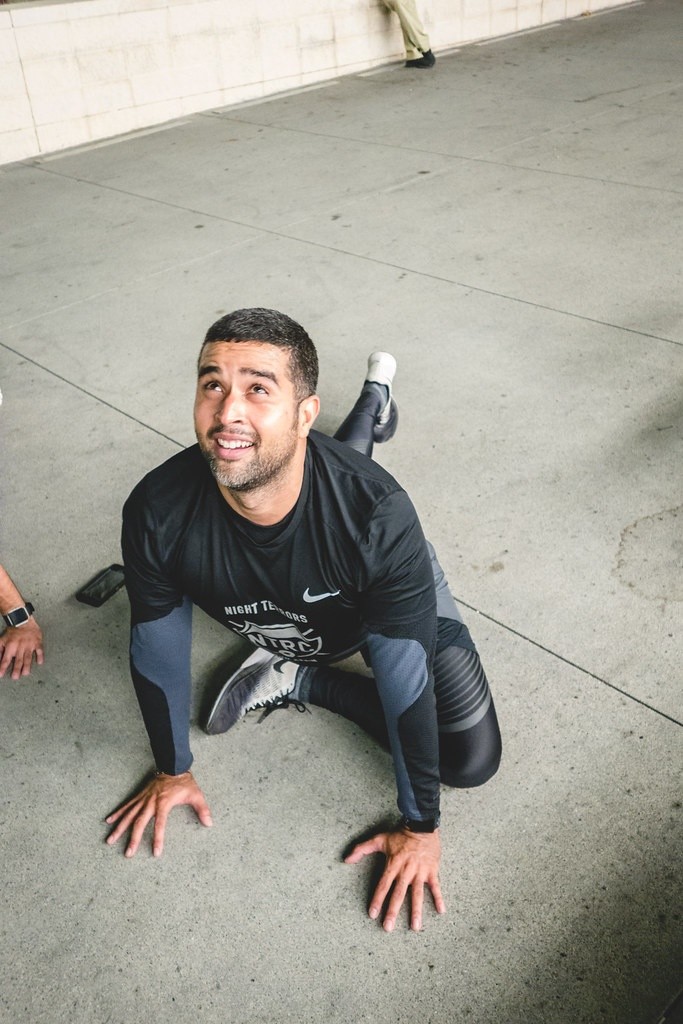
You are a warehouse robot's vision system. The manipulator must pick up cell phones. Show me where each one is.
[76,564,127,607]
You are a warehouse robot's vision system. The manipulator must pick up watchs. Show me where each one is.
[401,814,442,833]
[2,601,35,628]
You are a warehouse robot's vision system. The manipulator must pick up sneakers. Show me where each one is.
[205,647,309,737]
[365,352,398,444]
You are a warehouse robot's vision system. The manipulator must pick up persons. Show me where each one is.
[102,306,503,936]
[1,562,46,679]
